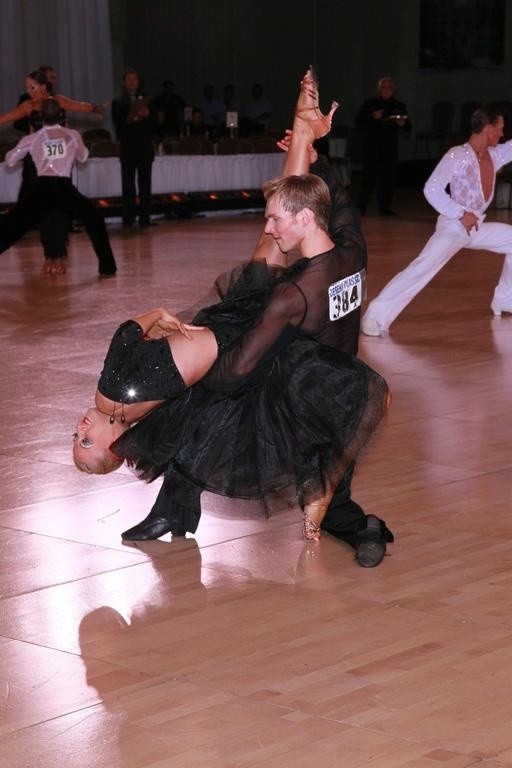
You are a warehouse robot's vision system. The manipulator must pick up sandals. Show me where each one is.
[41,260,66,275]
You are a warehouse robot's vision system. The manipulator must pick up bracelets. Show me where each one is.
[91,104,103,112]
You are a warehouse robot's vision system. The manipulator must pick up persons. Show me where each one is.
[75,64,389,549]
[351,75,412,213]
[1,102,117,279]
[110,66,160,227]
[154,80,272,136]
[14,63,59,137]
[358,103,511,338]
[1,70,106,273]
[119,94,393,571]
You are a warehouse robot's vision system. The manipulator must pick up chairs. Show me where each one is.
[0,119,287,161]
[415,100,483,153]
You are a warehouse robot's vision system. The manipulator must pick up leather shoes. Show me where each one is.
[139,219,160,226]
[122,214,135,225]
[359,204,367,216]
[98,267,119,275]
[379,207,397,218]
[360,315,380,337]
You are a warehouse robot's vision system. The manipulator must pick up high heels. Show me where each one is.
[356,509,387,567]
[295,64,340,141]
[120,509,188,541]
[490,298,512,317]
[287,477,329,544]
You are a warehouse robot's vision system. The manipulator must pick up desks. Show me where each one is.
[1,152,288,220]
